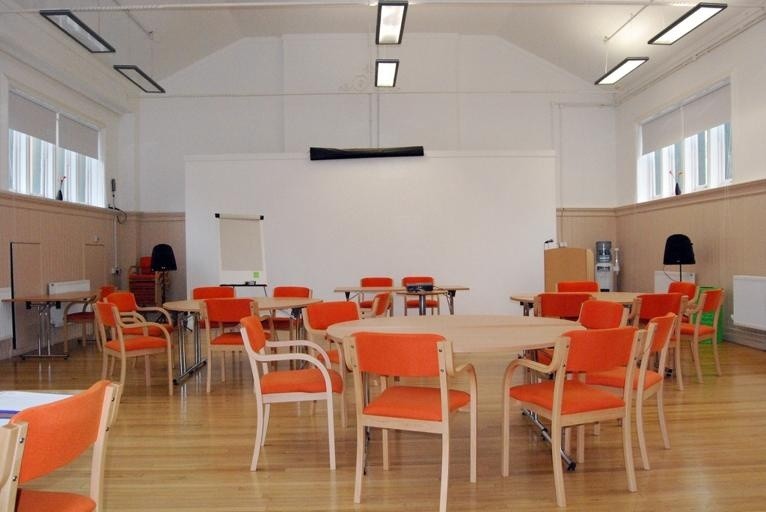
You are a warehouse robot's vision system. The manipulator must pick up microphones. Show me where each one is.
[544,239,554,243]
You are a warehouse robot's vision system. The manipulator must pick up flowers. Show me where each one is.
[55,174,66,191]
[669,168,685,183]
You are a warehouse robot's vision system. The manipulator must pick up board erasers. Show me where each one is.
[245,281,256,286]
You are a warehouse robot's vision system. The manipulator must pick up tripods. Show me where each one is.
[155,271,176,325]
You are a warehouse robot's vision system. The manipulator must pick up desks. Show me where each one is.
[1,292,97,359]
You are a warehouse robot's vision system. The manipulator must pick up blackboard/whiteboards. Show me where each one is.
[215,213,268,287]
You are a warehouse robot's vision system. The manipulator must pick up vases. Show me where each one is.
[676,182,682,195]
[54,190,63,201]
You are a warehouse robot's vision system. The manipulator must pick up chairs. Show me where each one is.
[92,302,174,398]
[2,377,125,512]
[125,256,168,307]
[102,291,177,371]
[163,255,726,511]
[62,282,118,358]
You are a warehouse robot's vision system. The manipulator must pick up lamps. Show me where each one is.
[149,244,178,325]
[594,17,650,86]
[375,58,402,89]
[645,1,729,48]
[374,1,411,46]
[663,232,698,281]
[111,18,170,96]
[37,2,117,55]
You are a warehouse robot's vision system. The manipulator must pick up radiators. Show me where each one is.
[730,273,766,332]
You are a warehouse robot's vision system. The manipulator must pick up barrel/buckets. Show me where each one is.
[595,240,613,264]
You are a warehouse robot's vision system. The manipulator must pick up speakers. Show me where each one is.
[663,234,695,264]
[150,244,177,271]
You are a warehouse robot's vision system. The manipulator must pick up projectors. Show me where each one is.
[407,283,434,292]
